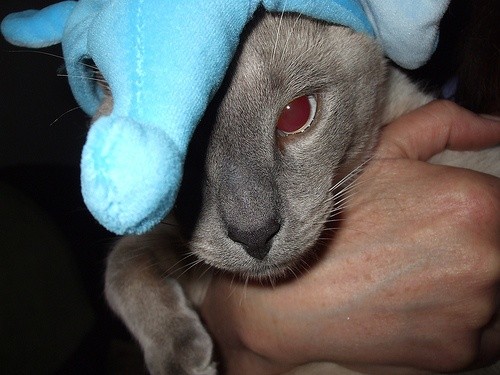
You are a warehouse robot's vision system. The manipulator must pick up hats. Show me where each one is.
[2,0,450,237]
